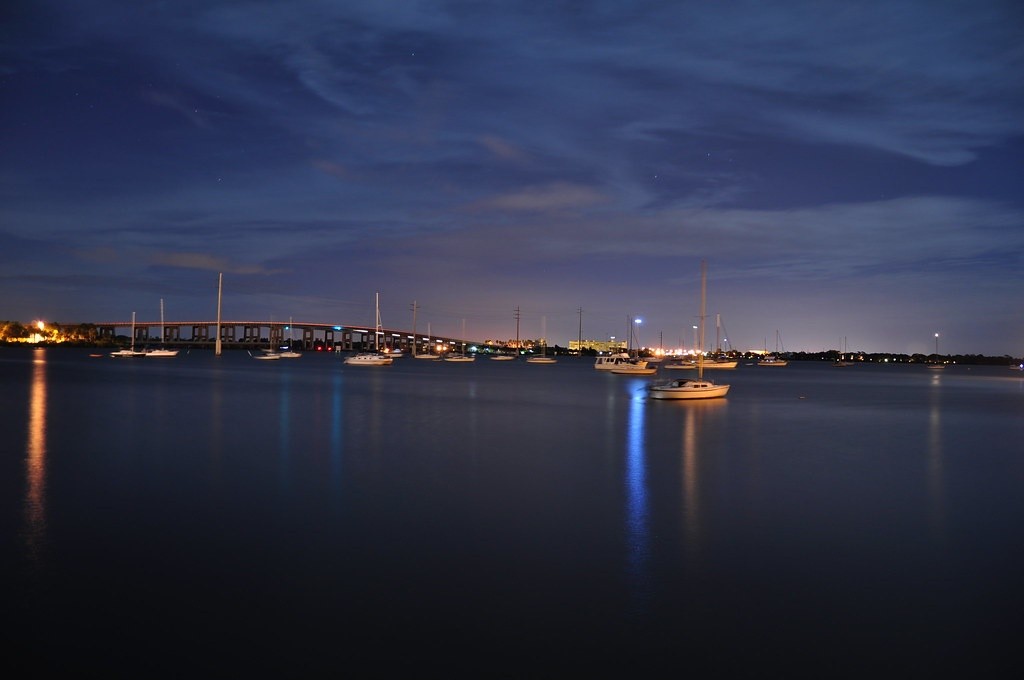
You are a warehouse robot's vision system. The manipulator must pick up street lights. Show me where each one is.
[935,333,938,358]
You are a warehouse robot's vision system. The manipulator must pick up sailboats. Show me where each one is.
[522,315,558,363]
[694,313,737,367]
[253,319,281,360]
[445,319,475,362]
[594,315,648,369]
[267,316,301,357]
[757,329,788,366]
[140,298,180,356]
[345,292,394,365]
[714,314,729,362]
[664,339,697,369]
[832,337,846,366]
[840,336,855,365]
[611,317,658,374]
[415,322,440,358]
[111,311,146,357]
[649,260,731,398]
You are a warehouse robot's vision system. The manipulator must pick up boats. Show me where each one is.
[384,353,404,357]
[88,353,103,357]
[1009,364,1024,369]
[491,356,514,360]
[644,357,662,361]
[927,365,946,369]
